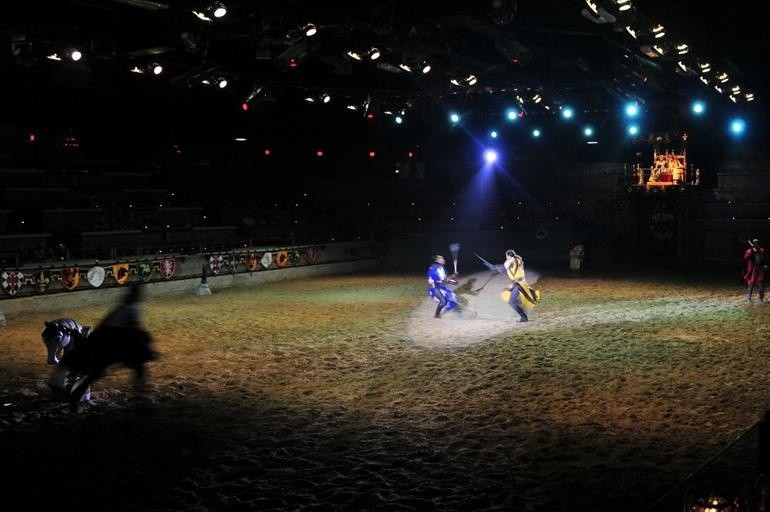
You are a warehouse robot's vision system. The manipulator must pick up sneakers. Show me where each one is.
[747,300,767,305]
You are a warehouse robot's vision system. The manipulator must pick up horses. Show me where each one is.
[41,318,96,404]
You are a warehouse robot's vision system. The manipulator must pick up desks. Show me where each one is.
[651,166,687,183]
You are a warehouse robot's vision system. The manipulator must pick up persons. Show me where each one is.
[742,238,767,302]
[490,249,541,322]
[66,282,159,413]
[426,254,461,318]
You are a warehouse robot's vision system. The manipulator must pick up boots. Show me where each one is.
[515,306,530,323]
[435,304,443,319]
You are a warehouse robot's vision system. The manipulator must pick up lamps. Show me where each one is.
[581,0,755,105]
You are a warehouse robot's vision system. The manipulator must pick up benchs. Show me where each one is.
[653,151,686,168]
